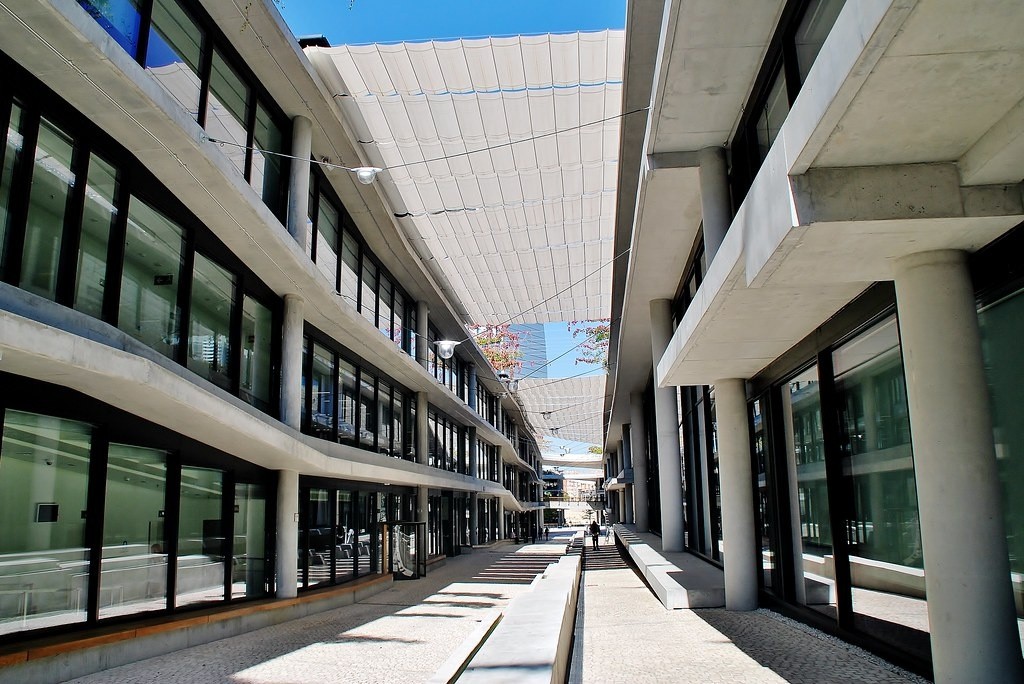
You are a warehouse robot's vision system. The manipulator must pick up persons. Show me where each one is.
[538,526,543,540]
[531,523,537,544]
[511,529,517,538]
[545,527,549,541]
[590,521,600,551]
[569,521,572,528]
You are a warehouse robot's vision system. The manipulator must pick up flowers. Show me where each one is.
[465,319,608,379]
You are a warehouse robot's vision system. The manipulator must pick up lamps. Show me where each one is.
[498,373,509,382]
[432,340,461,359]
[541,408,552,420]
[550,426,560,435]
[350,166,383,184]
